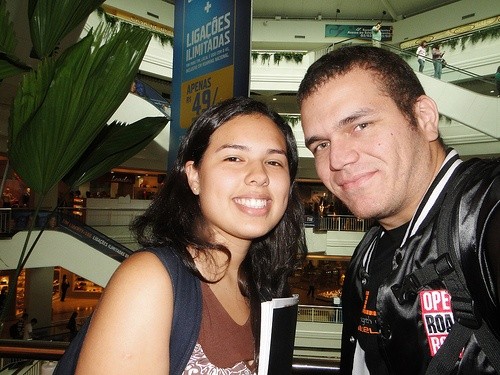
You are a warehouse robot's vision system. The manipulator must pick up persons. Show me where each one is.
[372,22,383,48]
[0,273,79,340]
[73,96,308,375]
[416,40,446,80]
[297,45,500,375]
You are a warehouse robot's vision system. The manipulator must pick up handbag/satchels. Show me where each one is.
[441,59,447,68]
[66,323,76,330]
[52,245,203,375]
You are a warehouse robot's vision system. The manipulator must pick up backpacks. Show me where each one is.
[9,321,23,338]
